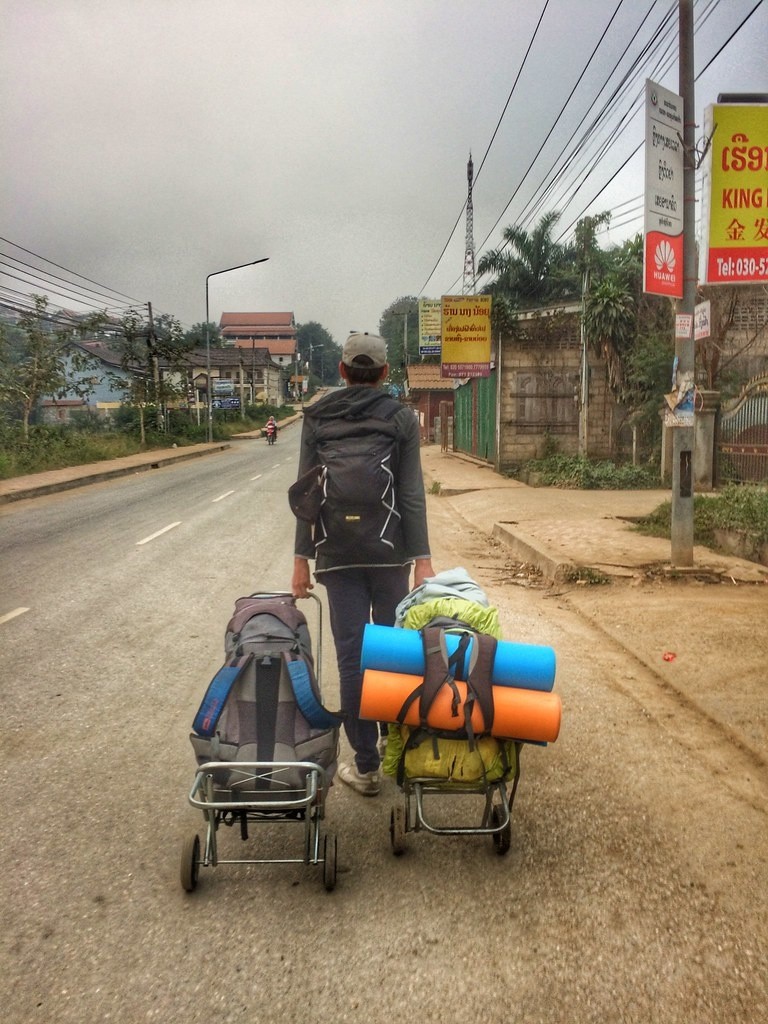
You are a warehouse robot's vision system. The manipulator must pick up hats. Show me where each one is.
[342,331,387,368]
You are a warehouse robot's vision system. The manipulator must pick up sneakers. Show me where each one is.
[379,736,388,761]
[337,762,380,795]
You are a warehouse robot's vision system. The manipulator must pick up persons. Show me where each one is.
[289,330,437,795]
[264,416,278,441]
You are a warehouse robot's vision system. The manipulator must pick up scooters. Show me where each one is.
[267,421,275,445]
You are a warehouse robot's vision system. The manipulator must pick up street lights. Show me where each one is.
[206,258,270,442]
[310,344,335,381]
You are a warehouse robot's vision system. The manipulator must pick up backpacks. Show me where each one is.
[189,593,342,802]
[315,398,405,556]
[382,568,518,787]
